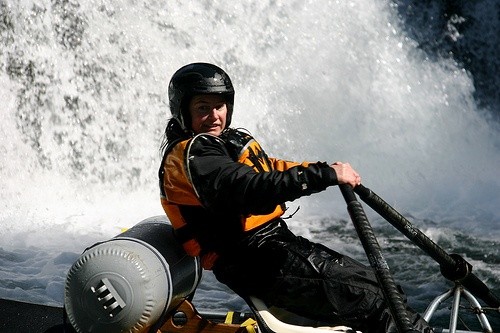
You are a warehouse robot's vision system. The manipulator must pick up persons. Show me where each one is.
[158,64,431,333]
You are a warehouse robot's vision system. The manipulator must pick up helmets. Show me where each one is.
[169,62,234,127]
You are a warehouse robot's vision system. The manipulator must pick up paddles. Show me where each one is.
[351,180,500,310]
[336,179,422,333]
